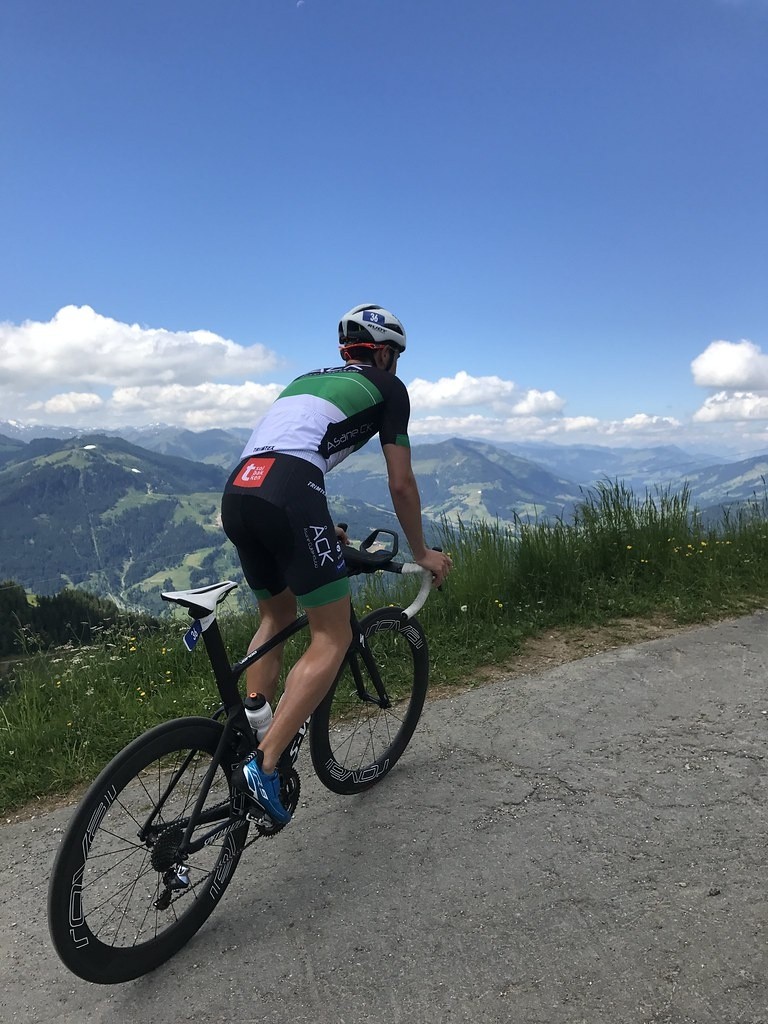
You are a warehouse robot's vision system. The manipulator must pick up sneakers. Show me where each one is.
[230,748,292,823]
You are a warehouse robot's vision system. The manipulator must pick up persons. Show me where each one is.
[221,304,454,820]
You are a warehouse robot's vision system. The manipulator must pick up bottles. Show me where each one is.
[244,693,273,743]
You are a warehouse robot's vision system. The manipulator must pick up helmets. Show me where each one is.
[338,303,406,353]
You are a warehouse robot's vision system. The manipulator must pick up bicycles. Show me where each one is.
[45,523,453,985]
